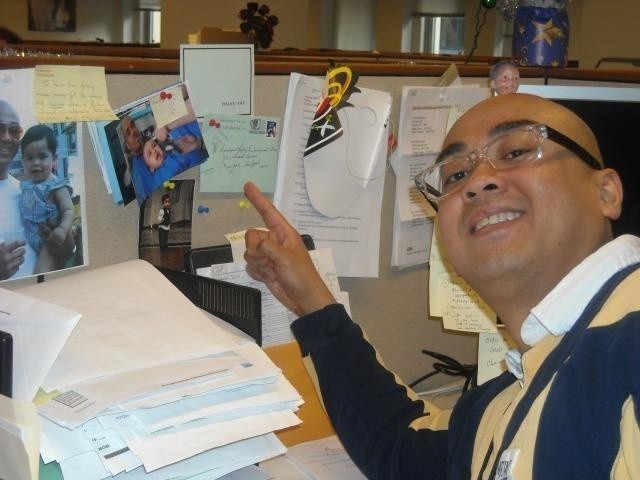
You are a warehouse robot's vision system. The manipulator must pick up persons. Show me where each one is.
[243,93,640,480]
[121,116,209,207]
[489,61,520,95]
[0,97,76,280]
[156,194,172,253]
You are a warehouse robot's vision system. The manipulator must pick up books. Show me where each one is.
[0,256,306,480]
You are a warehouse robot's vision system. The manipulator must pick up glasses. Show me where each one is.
[1,124,24,139]
[414,124,602,201]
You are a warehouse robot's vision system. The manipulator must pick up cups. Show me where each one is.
[2,227,25,247]
[514,7,569,66]
